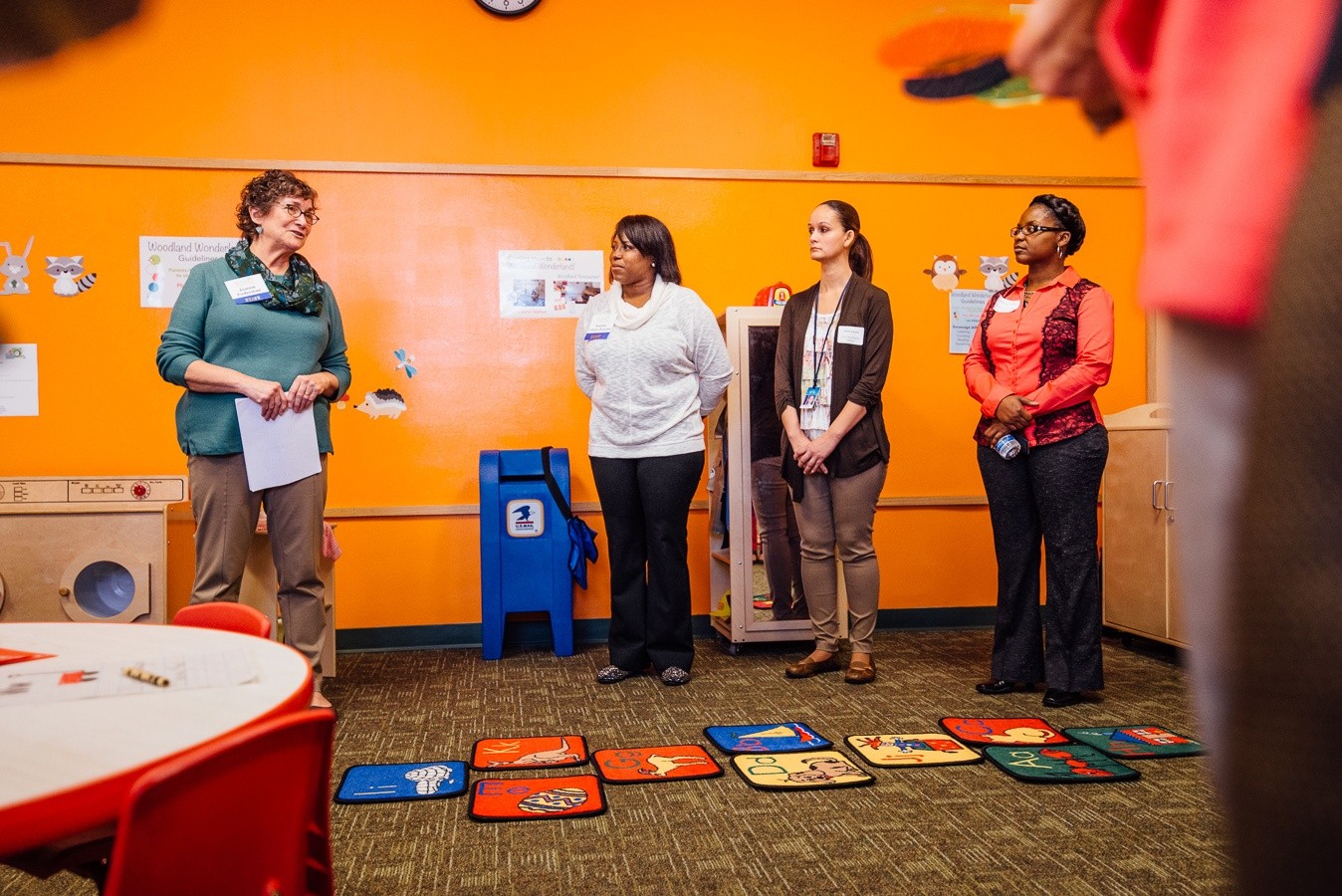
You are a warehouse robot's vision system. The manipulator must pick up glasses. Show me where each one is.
[1010,224,1068,238]
[268,197,320,225]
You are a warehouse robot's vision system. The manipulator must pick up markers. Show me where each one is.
[118,667,170,688]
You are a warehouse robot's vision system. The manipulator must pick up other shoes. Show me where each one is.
[785,647,843,678]
[596,661,638,684]
[658,666,692,685]
[846,648,878,683]
[309,691,339,723]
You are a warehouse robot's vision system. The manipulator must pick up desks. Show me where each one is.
[0,620,315,861]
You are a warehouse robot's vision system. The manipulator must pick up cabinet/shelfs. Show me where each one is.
[707,305,853,657]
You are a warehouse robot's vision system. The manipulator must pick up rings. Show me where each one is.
[304,392,311,396]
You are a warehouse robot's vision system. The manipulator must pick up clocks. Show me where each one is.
[475,0,542,17]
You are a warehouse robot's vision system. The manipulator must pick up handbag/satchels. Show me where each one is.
[566,515,599,592]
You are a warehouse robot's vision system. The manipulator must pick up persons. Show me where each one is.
[999,0,1342,896]
[770,199,891,682]
[156,169,350,719]
[962,194,1117,704]
[574,216,734,684]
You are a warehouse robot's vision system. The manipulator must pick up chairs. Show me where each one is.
[106,708,335,896]
[171,602,272,641]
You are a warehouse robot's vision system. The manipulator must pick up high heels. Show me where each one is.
[1042,686,1086,707]
[976,674,1042,694]
